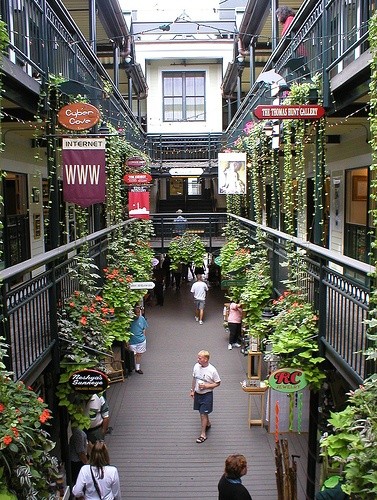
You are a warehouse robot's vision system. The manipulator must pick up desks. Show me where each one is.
[248,350,263,380]
[248,392,265,428]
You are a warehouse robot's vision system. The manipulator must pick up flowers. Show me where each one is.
[0,234,377,500]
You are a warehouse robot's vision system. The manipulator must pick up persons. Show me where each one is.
[128,305,148,374]
[275,5,306,57]
[162,257,194,291]
[81,393,110,443]
[72,440,121,500]
[218,454,252,500]
[193,260,207,276]
[154,268,164,305]
[69,428,89,495]
[228,301,243,349]
[174,209,187,230]
[191,350,221,443]
[191,274,209,324]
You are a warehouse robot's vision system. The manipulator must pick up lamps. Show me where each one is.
[236,34,246,62]
[270,56,318,105]
[125,55,133,63]
[99,115,110,135]
[159,25,170,31]
[59,81,90,95]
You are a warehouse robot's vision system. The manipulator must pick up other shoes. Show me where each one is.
[235,343,241,348]
[199,321,204,324]
[128,372,131,376]
[195,316,199,322]
[136,370,143,374]
[160,303,163,306]
[228,344,232,349]
[155,303,159,305]
[124,373,128,378]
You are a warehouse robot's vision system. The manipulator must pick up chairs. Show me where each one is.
[104,350,126,383]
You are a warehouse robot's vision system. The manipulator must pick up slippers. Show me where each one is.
[206,425,211,431]
[196,437,208,443]
